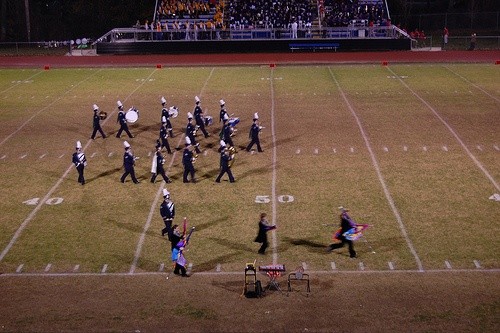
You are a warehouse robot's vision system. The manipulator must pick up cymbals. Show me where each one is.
[100,111,107,120]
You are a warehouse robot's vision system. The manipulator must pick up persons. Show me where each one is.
[148,95,238,183]
[245,113,264,152]
[115,100,134,138]
[442,27,448,43]
[470,33,477,43]
[72,141,87,185]
[253,213,277,253]
[91,104,107,139]
[134,0,426,49]
[160,188,175,240]
[167,224,188,277]
[329,207,358,258]
[120,140,140,184]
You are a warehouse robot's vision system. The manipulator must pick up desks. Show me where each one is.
[259,264,285,295]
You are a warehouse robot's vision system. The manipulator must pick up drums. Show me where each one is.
[168,106,178,118]
[228,117,240,127]
[204,116,213,126]
[125,107,139,123]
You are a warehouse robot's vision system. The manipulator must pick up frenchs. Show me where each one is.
[220,146,236,168]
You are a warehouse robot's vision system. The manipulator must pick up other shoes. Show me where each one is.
[150,179,172,184]
[161,229,164,236]
[120,179,140,184]
[77,177,86,186]
[183,179,200,183]
[168,134,176,138]
[173,271,190,277]
[168,150,173,154]
[194,133,212,138]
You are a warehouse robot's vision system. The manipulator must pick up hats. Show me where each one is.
[252,112,259,119]
[92,104,99,111]
[219,100,225,105]
[195,96,200,102]
[161,115,166,122]
[220,140,227,148]
[123,141,131,149]
[187,112,193,118]
[162,189,170,196]
[75,140,83,149]
[184,135,192,145]
[223,114,229,120]
[117,101,122,108]
[161,97,166,103]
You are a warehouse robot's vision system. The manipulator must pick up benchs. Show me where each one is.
[289,43,340,54]
